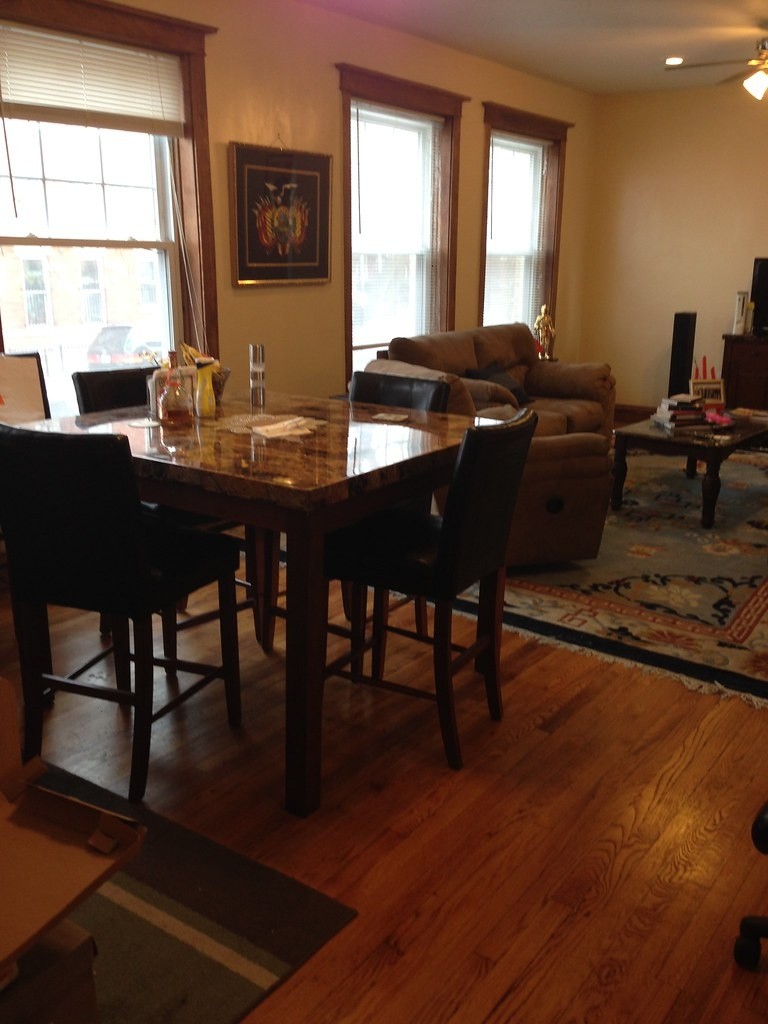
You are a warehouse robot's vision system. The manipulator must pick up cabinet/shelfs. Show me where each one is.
[719,335,768,411]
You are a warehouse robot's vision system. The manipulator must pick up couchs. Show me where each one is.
[378,320,617,451]
[363,356,614,569]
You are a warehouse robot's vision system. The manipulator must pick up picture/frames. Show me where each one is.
[227,140,338,288]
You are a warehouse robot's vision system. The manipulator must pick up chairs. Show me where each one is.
[324,407,537,771]
[73,364,173,418]
[261,371,451,672]
[2,422,249,803]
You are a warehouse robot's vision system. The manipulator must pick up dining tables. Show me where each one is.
[13,397,505,817]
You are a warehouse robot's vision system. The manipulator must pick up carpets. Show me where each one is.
[449,456,768,704]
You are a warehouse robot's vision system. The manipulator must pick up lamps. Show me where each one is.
[743,69,768,100]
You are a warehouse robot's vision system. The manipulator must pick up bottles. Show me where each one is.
[157,351,195,427]
[195,357,216,418]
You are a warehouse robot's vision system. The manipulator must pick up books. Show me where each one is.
[649,394,710,431]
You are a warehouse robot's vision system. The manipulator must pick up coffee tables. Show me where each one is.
[613,411,768,529]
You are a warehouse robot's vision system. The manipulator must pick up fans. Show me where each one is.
[664,40,768,100]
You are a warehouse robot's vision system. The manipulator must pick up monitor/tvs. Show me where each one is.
[750,257,768,327]
